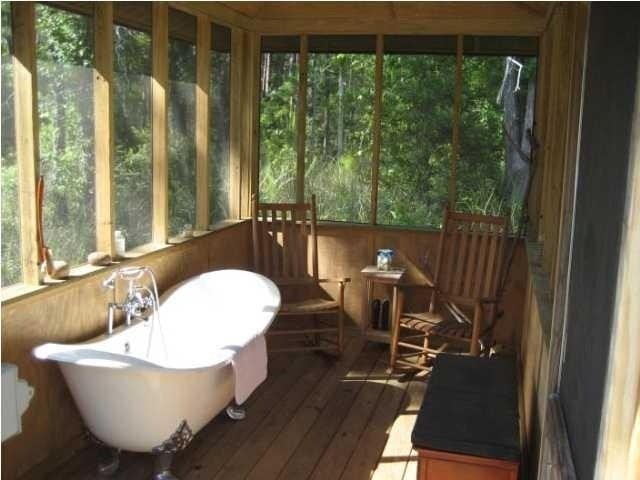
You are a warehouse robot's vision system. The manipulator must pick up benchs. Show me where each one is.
[410,351,521,480]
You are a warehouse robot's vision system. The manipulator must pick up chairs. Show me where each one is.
[386,205,511,388]
[252,192,348,370]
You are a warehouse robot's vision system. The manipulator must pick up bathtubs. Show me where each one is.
[29,267,283,454]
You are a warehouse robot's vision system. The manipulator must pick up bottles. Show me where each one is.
[115,230,126,254]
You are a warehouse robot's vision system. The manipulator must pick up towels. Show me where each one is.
[225,330,268,405]
